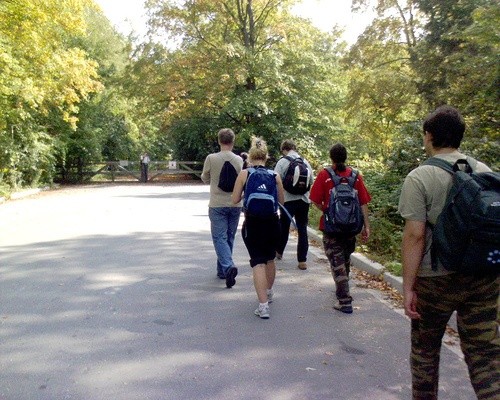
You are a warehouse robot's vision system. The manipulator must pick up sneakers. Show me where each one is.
[255,305,270,318]
[334,302,353,313]
[267,293,272,302]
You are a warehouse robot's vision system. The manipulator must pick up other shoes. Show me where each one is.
[298,262,307,270]
[276,251,282,260]
[217,273,225,279]
[226,267,238,288]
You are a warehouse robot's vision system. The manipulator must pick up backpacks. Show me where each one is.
[243,169,278,214]
[323,166,361,227]
[280,156,310,196]
[422,157,500,277]
[218,154,238,192]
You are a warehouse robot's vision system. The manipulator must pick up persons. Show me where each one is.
[309,144,371,313]
[140,152,150,182]
[274,139,314,270]
[394,106,500,400]
[200,128,243,288]
[231,136,284,319]
